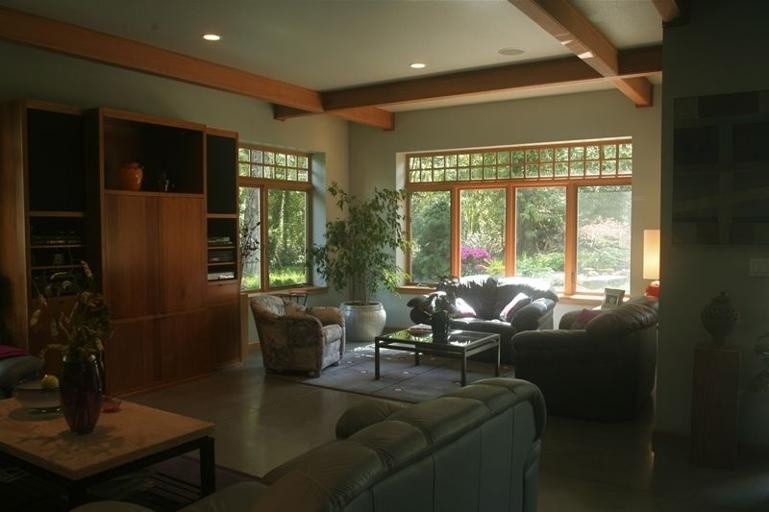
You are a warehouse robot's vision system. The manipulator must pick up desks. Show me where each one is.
[693,340,742,471]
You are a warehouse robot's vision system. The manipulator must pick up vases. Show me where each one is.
[117,161,145,193]
[57,371,102,434]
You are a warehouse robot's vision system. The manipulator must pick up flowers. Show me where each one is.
[34,256,114,399]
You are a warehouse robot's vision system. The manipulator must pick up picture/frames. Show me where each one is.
[602,288,626,308]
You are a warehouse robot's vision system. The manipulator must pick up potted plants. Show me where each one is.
[419,275,460,340]
[312,178,416,342]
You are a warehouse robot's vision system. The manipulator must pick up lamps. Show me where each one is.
[642,228,661,299]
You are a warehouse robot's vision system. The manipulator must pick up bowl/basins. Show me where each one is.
[408,326,433,337]
[101,399,122,412]
[11,379,64,413]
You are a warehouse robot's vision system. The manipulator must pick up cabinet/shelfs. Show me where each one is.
[202,127,243,373]
[1,98,105,393]
[82,106,209,398]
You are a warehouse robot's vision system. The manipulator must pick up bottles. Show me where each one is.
[119,160,143,190]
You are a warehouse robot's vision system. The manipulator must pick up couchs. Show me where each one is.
[510,300,660,423]
[250,294,346,378]
[74,375,549,512]
[408,272,560,366]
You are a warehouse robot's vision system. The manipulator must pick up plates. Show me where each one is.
[10,408,66,420]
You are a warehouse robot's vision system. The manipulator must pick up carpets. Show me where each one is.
[2,452,260,512]
[289,340,516,405]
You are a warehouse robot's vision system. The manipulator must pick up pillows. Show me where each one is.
[569,305,601,330]
[450,298,478,318]
[498,291,531,322]
